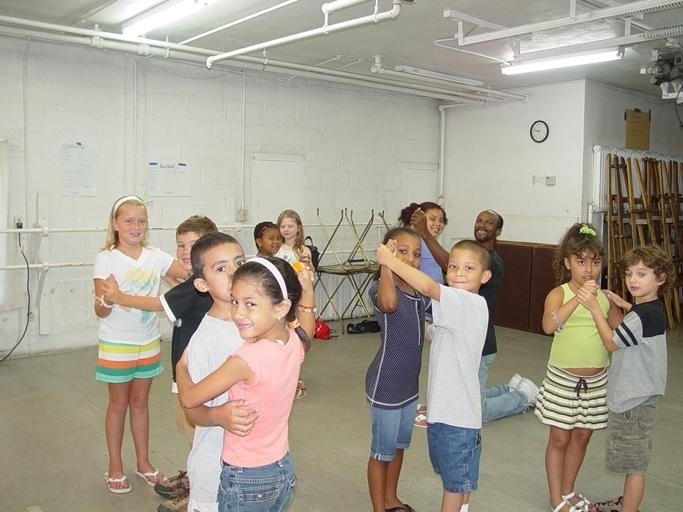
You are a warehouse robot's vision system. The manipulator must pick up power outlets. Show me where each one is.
[14,216,23,229]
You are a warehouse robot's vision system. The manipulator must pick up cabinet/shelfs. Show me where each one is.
[491,241,572,338]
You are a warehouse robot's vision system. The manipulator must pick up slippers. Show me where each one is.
[415,403,426,413]
[413,413,427,429]
[385,504,412,512]
[104,472,131,494]
[134,467,167,488]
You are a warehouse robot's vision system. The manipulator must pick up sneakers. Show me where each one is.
[508,373,521,391]
[594,496,640,512]
[154,471,189,498]
[157,491,189,512]
[516,377,539,408]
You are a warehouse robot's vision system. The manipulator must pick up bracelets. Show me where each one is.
[296,303,318,313]
[95,294,115,309]
[549,310,564,333]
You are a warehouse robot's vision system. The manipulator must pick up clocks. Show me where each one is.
[530,121,549,142]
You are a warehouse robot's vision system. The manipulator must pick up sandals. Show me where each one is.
[296,379,305,391]
[561,492,597,512]
[293,389,306,400]
[550,500,585,512]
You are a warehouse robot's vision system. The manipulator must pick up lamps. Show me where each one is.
[499,45,624,77]
[122,0,204,36]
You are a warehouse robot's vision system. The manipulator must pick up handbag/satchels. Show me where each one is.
[314,319,330,339]
[346,320,380,334]
[304,236,319,267]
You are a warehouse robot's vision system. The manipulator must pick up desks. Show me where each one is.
[311,207,404,334]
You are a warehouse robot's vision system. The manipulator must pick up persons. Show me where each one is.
[472,210,541,422]
[102,212,218,512]
[539,220,623,512]
[173,257,314,512]
[396,199,448,427]
[375,239,490,511]
[173,231,245,512]
[253,220,281,257]
[576,244,672,512]
[90,196,188,494]
[359,228,432,512]
[277,208,315,401]
